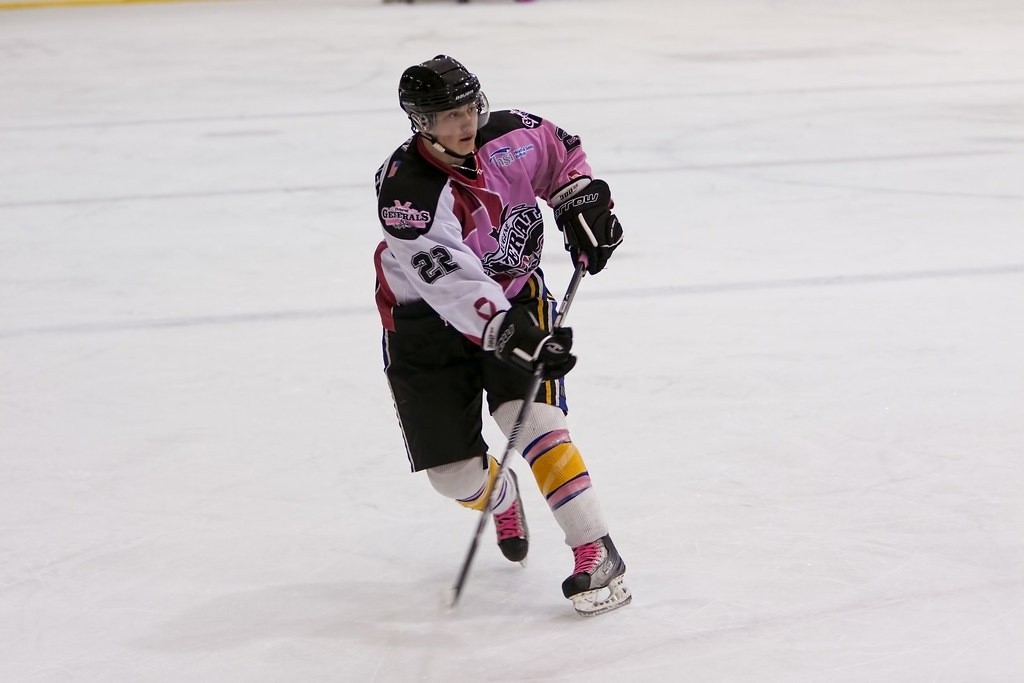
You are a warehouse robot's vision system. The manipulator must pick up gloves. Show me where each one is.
[484,304,577,381]
[548,175,625,276]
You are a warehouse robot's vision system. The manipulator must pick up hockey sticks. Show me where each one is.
[443,251,590,612]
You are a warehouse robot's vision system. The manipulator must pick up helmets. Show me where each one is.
[399,55,481,113]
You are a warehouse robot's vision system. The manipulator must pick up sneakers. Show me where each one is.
[562,533,633,617]
[457,456,531,566]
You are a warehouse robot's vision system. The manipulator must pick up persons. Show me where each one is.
[374,53,632,618]
